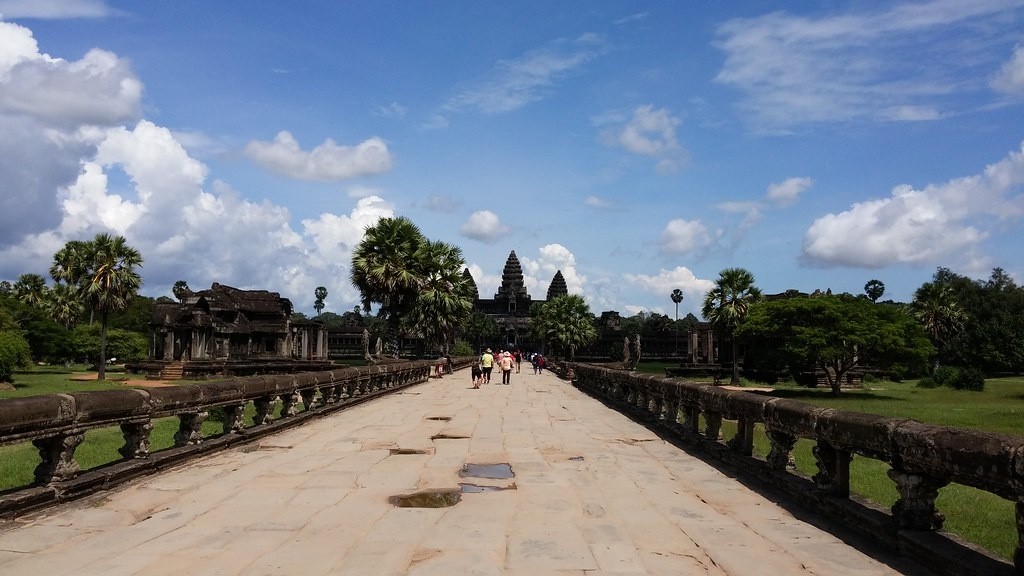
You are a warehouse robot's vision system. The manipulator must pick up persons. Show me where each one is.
[471,357,482,389]
[447,355,454,375]
[491,349,544,384]
[482,348,494,384]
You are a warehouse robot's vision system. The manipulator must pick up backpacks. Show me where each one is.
[516,352,521,362]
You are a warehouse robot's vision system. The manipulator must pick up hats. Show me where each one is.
[486,348,492,352]
[501,350,503,352]
[535,353,538,355]
[504,351,510,356]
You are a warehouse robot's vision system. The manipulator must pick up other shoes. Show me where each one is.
[484,378,487,384]
[539,372,541,374]
[487,381,489,384]
[535,372,536,375]
[503,381,505,384]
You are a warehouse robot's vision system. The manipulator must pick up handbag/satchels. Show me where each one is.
[533,362,536,365]
